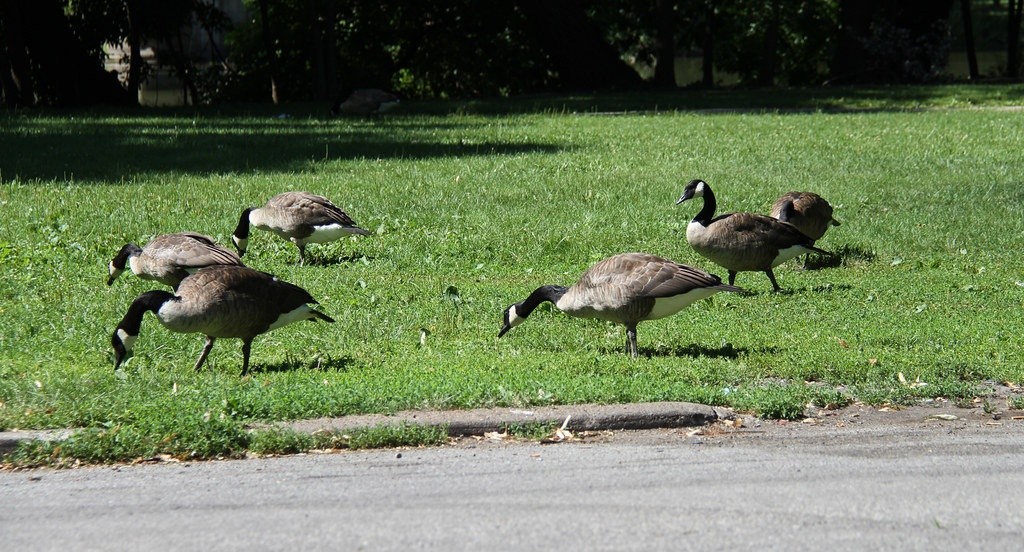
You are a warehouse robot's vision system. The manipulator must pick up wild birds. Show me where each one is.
[108,190,374,376]
[498,253,747,359]
[675,179,842,294]
